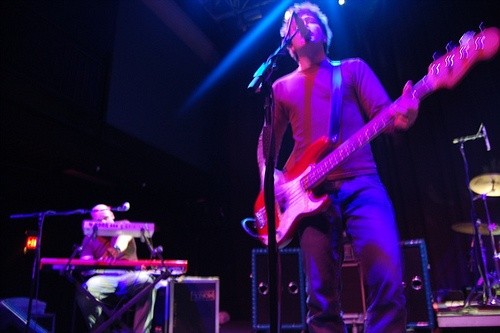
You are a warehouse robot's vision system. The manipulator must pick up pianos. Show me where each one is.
[33,255,189,276]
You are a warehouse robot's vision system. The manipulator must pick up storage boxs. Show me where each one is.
[148,276,219,333]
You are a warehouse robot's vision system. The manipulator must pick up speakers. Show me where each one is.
[0,299,49,333]
[252,247,306,330]
[401,239,434,327]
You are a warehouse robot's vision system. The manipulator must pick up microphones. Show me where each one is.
[482,126,490,151]
[292,12,311,41]
[123,202,130,211]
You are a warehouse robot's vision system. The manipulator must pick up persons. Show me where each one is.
[258,4,419,333]
[79,204,157,333]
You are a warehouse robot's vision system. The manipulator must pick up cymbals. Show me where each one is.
[450,221,499,236]
[466,173,499,198]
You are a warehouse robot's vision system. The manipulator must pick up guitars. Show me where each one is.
[255,23,499,248]
